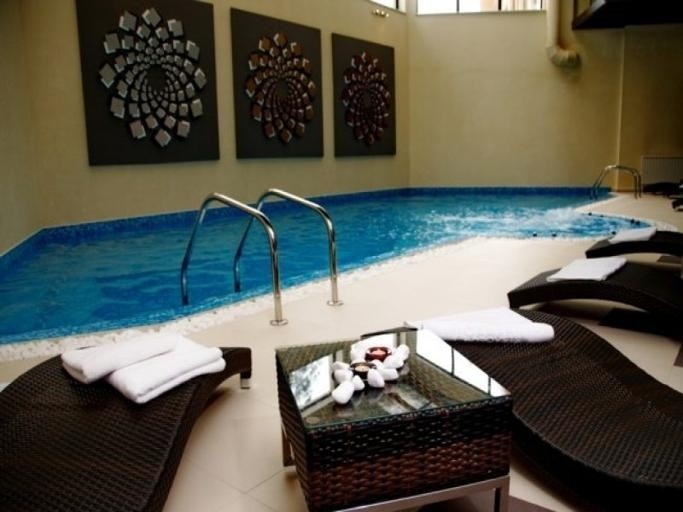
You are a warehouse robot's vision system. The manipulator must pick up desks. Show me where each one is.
[274,327,514,512]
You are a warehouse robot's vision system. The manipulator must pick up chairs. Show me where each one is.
[447,309,682,512]
[0,345,251,511]
[507,262,680,364]
[584,229,681,261]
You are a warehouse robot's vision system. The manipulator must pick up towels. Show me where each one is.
[59,333,226,404]
[411,307,555,344]
